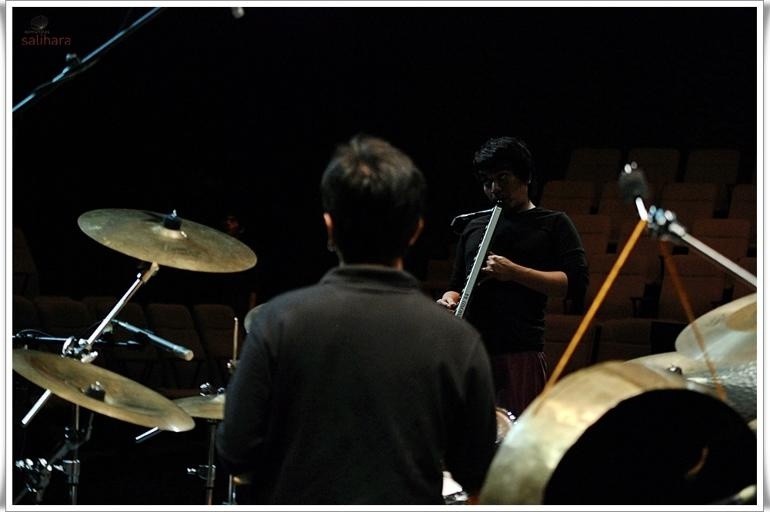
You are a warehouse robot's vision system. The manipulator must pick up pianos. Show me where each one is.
[450,200,504,319]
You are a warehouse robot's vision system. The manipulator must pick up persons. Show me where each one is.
[220,209,258,325]
[216,136,497,505]
[435,136,588,417]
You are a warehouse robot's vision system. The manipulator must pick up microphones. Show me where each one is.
[113,321,195,361]
[232,7,245,18]
[617,172,649,221]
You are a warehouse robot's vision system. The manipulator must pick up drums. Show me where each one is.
[480,358,756,504]
[441,407,516,477]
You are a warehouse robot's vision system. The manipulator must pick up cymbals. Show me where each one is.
[78,208,258,273]
[626,352,757,421]
[172,394,226,420]
[675,294,758,366]
[12,349,196,432]
